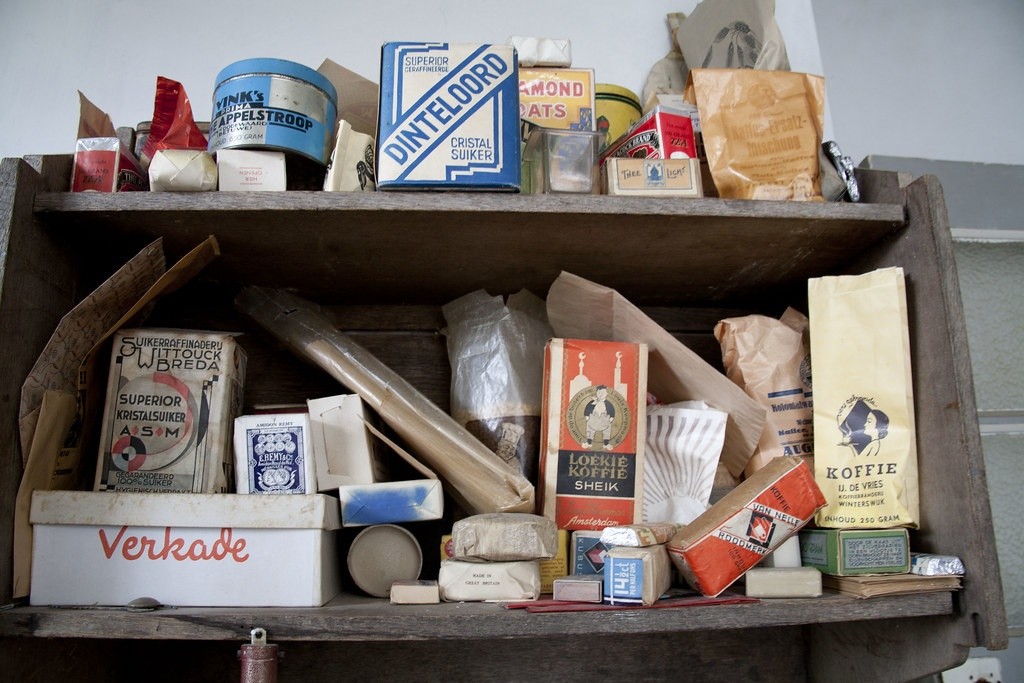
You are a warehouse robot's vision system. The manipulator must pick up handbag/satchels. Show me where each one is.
[666,0,845,201]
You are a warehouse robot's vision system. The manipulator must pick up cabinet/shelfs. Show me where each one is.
[1,121,1009,683]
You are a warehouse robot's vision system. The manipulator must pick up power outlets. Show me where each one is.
[942,657,1004,683]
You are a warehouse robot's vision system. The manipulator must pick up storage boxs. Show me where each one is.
[25,0,920,608]
